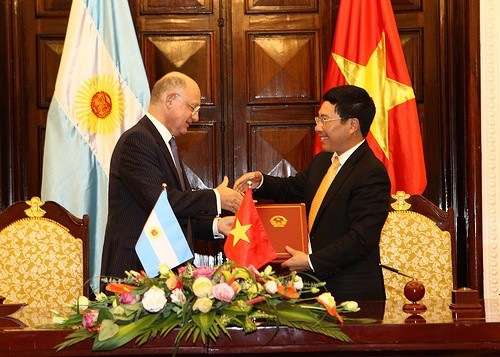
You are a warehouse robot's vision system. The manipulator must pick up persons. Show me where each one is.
[99,71,244,297]
[234,85,391,300]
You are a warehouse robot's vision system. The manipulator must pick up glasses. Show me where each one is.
[176,94,201,116]
[315,116,344,127]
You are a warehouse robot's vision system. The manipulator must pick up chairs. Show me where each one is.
[0,198,89,331]
[379,190,457,324]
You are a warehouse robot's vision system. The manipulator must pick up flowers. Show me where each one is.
[51,258,379,353]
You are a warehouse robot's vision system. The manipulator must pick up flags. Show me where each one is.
[314,0,427,195]
[224,189,277,270]
[41,0,150,295]
[135,191,193,278]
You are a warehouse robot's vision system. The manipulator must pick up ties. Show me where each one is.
[170,137,195,253]
[307,156,341,233]
